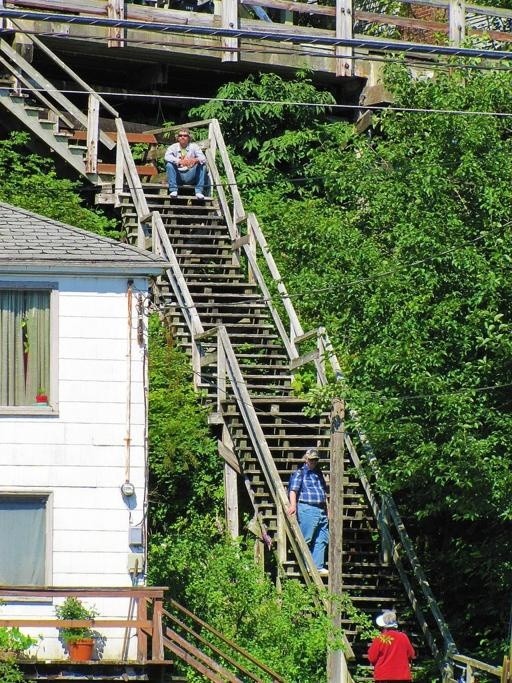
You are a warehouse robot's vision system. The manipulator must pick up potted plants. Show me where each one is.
[54,595,100,661]
[0,625,38,660]
[36,387,47,403]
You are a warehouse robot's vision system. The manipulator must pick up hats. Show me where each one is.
[305,448,321,459]
[375,611,399,628]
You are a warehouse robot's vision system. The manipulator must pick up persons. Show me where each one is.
[164,127,208,201]
[287,446,332,576]
[366,612,417,682]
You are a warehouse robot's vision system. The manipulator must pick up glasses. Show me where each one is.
[178,134,188,137]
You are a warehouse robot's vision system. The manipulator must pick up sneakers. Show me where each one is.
[196,193,204,199]
[170,191,177,197]
[317,567,329,574]
